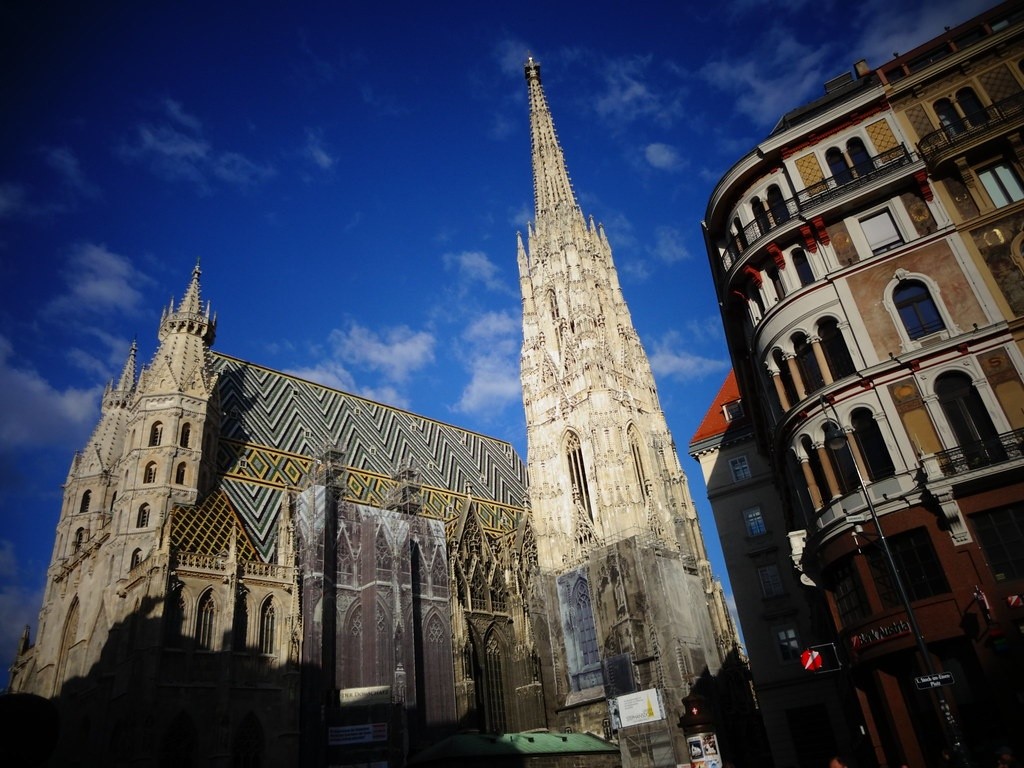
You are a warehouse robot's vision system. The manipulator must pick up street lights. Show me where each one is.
[530,648,548,729]
[820,395,972,767]
